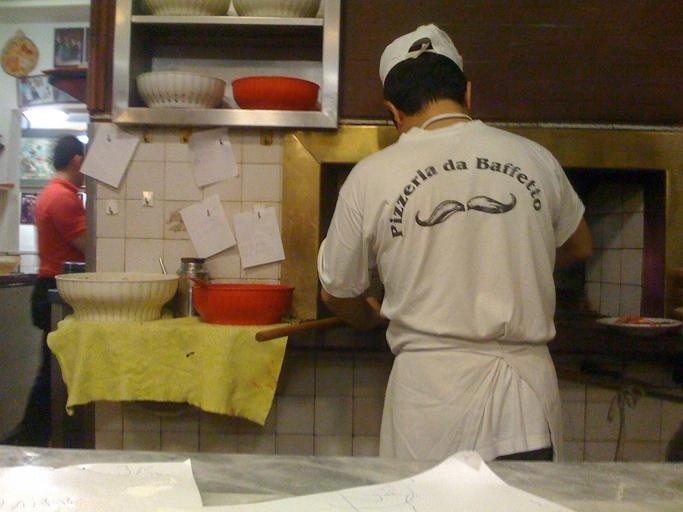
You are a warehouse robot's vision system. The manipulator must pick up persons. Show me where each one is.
[22,134,86,449]
[313,22,592,462]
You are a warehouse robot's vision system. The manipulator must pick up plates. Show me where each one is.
[595,317,683,335]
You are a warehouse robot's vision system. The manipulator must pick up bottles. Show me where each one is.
[175,256,206,319]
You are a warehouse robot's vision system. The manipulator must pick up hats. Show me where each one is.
[379,23,464,88]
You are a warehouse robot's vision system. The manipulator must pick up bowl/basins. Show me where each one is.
[52,272,180,320]
[138,0,323,19]
[229,75,320,112]
[191,284,294,325]
[133,70,227,111]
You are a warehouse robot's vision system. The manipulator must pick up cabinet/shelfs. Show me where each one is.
[110,0,342,133]
